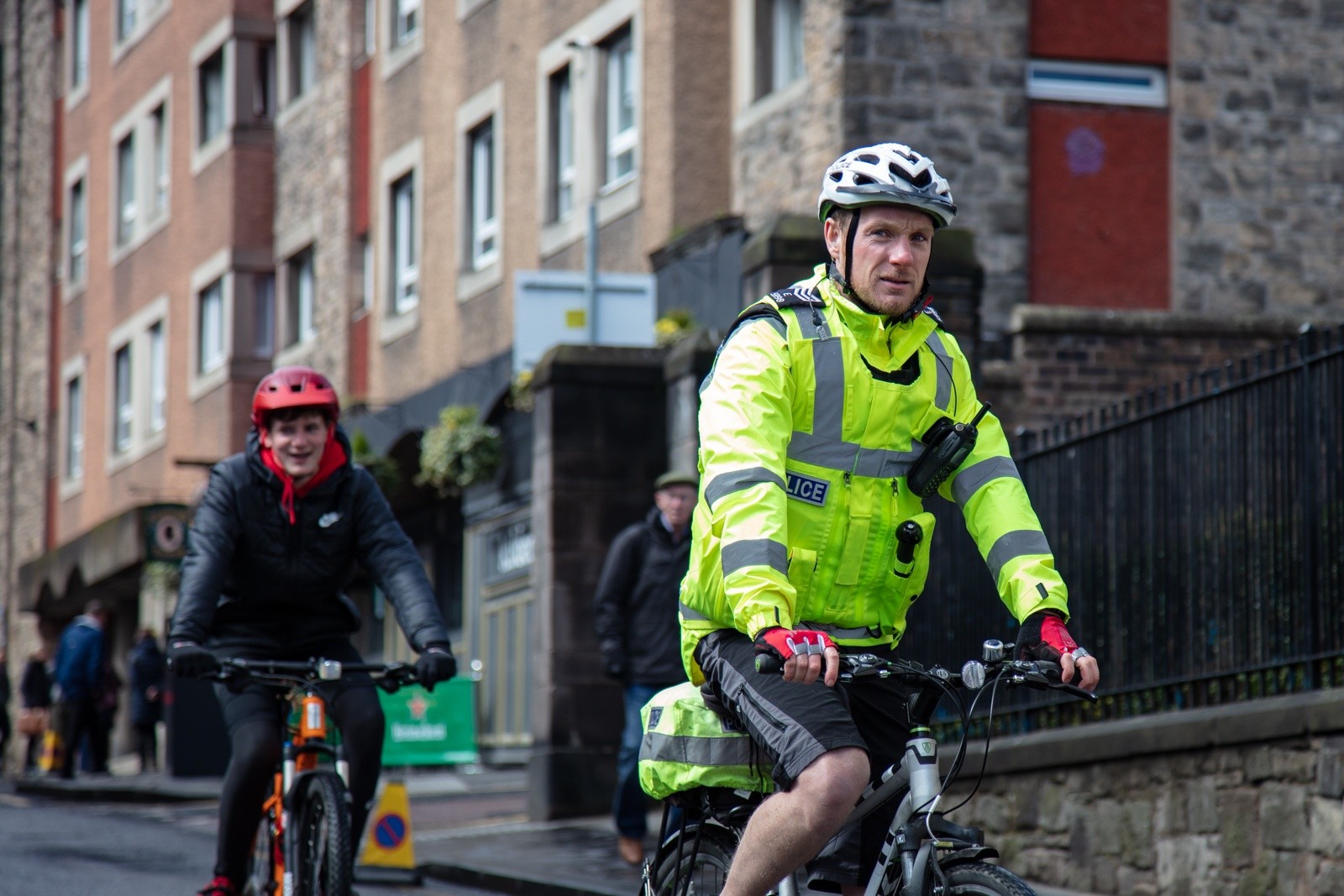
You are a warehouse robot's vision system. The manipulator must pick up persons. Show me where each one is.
[0,605,164,777]
[171,367,457,896]
[596,473,698,865]
[679,143,1099,896]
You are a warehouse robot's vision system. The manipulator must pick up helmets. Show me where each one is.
[252,370,341,425]
[818,143,957,227]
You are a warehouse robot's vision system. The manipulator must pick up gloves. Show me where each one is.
[167,637,222,675]
[415,646,456,693]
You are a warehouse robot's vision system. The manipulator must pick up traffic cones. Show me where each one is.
[352,785,422,887]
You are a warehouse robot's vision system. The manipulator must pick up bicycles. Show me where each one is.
[169,647,415,896]
[639,638,1099,896]
